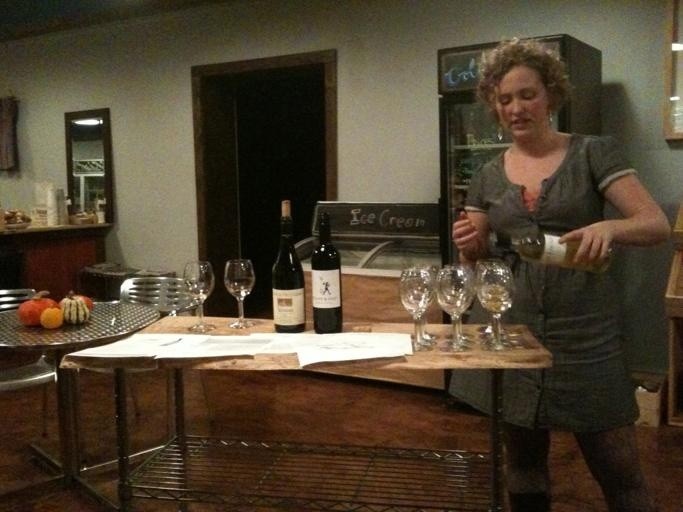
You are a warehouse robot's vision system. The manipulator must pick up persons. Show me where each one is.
[451,39,671,512]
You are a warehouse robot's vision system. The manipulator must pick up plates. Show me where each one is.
[3,223,31,229]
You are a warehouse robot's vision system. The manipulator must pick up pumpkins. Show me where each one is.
[40,304,64,329]
[18,290,61,326]
[57,295,90,324]
[59,296,93,312]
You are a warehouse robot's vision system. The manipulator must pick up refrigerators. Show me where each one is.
[291,236,446,392]
[435,32,602,423]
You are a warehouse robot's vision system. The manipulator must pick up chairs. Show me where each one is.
[93,277,215,439]
[0,289,79,439]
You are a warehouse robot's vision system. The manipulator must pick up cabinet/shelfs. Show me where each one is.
[665,201,683,430]
[63,315,554,512]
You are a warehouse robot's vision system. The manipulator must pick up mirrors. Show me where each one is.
[63,108,112,224]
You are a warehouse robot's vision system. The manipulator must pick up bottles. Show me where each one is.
[490,231,612,275]
[272,200,306,333]
[310,213,343,335]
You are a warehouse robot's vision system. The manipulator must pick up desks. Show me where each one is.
[0,224,112,302]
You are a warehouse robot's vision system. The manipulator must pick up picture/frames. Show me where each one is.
[664,0,683,147]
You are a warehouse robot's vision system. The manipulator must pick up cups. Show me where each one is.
[476,259,516,351]
[182,260,216,333]
[398,264,439,353]
[223,259,255,329]
[46,188,59,227]
[58,188,69,224]
[435,263,477,352]
